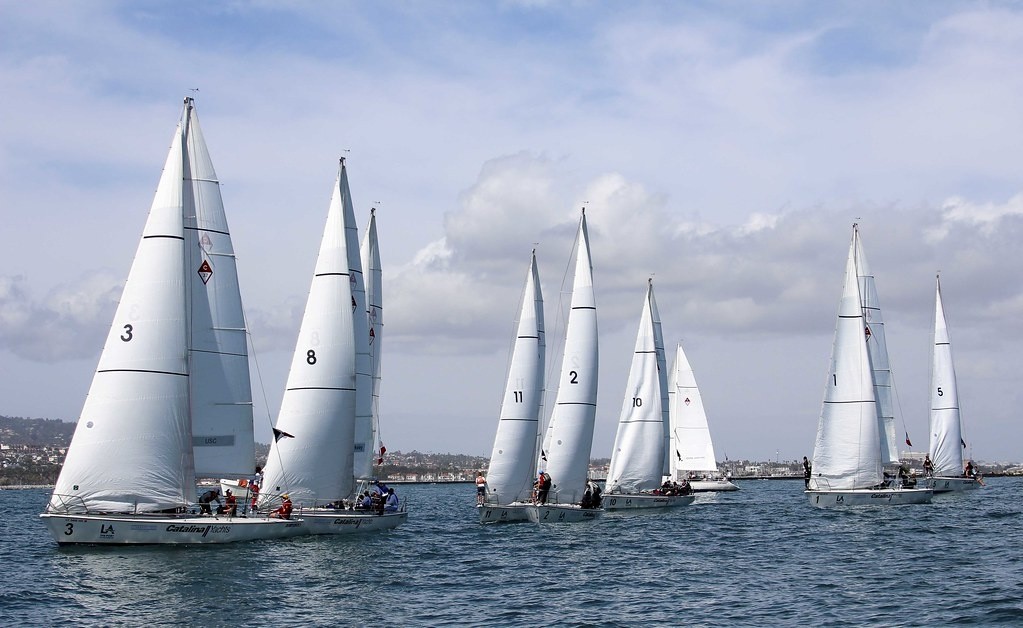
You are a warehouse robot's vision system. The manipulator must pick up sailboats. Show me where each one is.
[475,199,607,523]
[218,143,411,535]
[600,269,697,507]
[38,89,308,543]
[802,217,935,506]
[662,338,744,493]
[922,269,986,491]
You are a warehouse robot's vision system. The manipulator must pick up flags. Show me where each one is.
[378,439,386,465]
[374,474,388,493]
[272,427,295,442]
[542,450,547,461]
[905,433,912,446]
[961,439,966,448]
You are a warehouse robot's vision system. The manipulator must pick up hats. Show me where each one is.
[225,489,232,494]
[214,488,220,495]
[280,494,289,499]
[538,469,544,475]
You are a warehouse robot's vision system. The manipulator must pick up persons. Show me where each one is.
[802,456,812,487]
[898,466,917,488]
[198,488,220,515]
[654,479,691,496]
[965,462,985,486]
[476,473,486,505]
[269,494,292,519]
[582,480,602,508]
[222,489,237,515]
[923,456,934,475]
[533,471,550,504]
[884,474,896,487]
[248,482,259,511]
[330,488,398,512]
